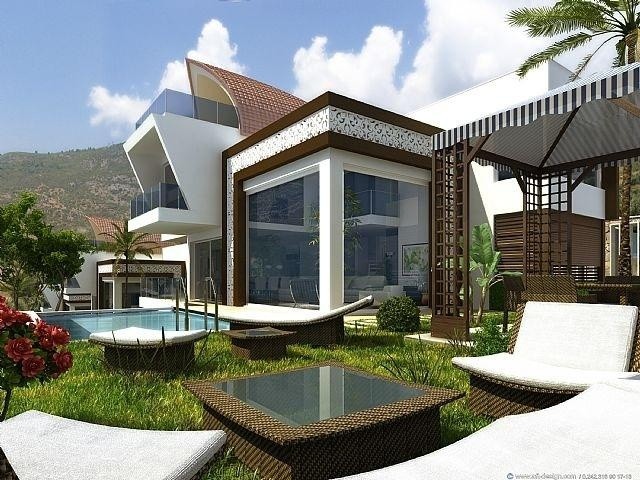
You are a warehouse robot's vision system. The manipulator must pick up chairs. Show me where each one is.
[247,270,420,309]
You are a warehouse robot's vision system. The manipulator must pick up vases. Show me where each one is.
[419,293,430,306]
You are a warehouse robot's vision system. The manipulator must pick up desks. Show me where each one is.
[577,279,633,307]
[220,323,295,363]
[172,359,467,480]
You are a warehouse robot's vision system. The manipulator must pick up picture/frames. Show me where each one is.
[399,242,428,276]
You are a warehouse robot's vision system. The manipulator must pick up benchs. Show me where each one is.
[448,299,640,418]
[602,275,640,307]
[217,293,377,351]
[526,273,597,303]
[89,324,212,371]
[0,404,227,480]
[321,370,640,480]
